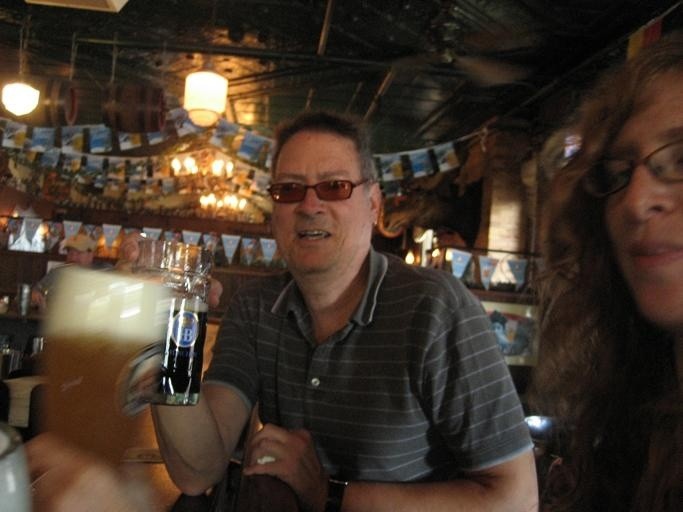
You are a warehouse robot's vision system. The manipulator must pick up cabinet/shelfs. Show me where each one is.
[0,200,542,404]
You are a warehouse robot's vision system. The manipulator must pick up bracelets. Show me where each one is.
[323,474,346,512]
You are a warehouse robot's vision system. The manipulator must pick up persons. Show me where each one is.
[532,38,683,512]
[130,109,539,512]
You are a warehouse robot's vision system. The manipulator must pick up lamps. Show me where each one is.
[1,2,41,117]
[183,0,229,128]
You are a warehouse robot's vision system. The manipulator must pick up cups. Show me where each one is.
[134,238,212,407]
[33,262,170,478]
[15,283,31,318]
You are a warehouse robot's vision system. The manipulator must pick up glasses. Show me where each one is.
[268,175,375,203]
[61,234,98,254]
[579,136,683,192]
